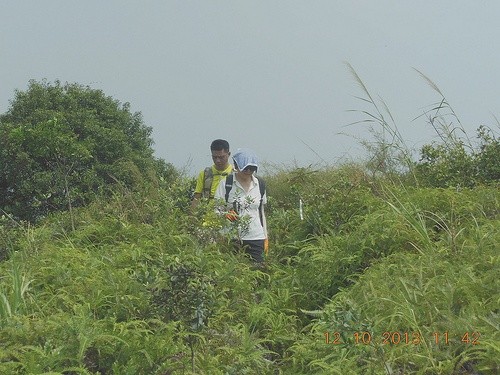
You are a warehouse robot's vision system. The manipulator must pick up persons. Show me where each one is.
[214,150,268,274]
[189,139,235,239]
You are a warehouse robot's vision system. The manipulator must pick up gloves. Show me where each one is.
[224,210,237,222]
[263,239,269,256]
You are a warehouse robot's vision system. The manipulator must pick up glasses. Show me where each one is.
[239,167,255,172]
